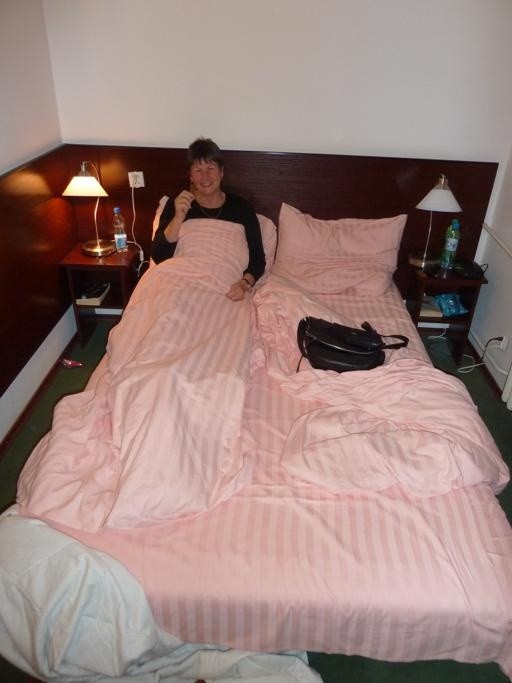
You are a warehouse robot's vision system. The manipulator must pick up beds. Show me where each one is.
[19,256,511,662]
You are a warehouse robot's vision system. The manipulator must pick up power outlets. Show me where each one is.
[128,171,145,188]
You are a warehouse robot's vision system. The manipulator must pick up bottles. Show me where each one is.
[441,218,460,269]
[113,208,128,255]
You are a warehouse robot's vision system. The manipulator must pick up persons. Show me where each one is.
[151,139,266,301]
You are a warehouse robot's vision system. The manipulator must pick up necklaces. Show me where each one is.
[195,190,223,218]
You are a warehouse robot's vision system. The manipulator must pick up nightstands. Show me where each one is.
[59,242,139,350]
[406,258,488,366]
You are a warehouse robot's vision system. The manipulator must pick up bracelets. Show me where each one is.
[242,278,251,288]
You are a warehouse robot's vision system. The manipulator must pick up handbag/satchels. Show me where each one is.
[304,316,385,373]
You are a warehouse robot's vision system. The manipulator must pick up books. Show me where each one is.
[420,295,442,318]
[76,280,111,306]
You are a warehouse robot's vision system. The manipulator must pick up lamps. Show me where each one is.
[60,160,114,258]
[407,173,464,268]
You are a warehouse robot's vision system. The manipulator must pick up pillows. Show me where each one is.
[151,195,408,277]
